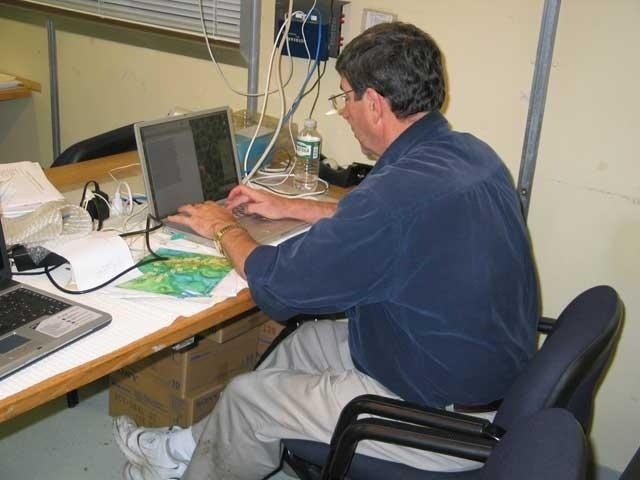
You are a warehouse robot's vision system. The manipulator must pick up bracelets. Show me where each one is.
[212,223,249,256]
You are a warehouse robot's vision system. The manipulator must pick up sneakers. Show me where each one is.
[112,412,190,480]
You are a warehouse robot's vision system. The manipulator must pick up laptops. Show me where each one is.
[0,216,112,382]
[134,105,314,255]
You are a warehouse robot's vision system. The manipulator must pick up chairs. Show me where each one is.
[48,121,144,168]
[327,408,589,480]
[282,285,625,480]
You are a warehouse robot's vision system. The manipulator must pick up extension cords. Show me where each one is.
[77,199,150,230]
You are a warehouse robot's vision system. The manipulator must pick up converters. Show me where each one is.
[87,192,108,218]
[12,245,69,273]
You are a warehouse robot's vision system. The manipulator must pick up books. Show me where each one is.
[0,160,236,303]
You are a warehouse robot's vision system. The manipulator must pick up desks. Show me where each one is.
[0,73,32,102]
[0,149,357,425]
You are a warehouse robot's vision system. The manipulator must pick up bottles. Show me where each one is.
[291,120,322,196]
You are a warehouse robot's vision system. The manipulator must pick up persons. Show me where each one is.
[107,18,541,480]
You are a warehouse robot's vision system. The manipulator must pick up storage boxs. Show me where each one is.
[108,319,284,429]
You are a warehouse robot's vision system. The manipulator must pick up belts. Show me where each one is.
[445,397,507,415]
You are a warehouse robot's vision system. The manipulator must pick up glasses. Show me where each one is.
[327,86,369,115]
